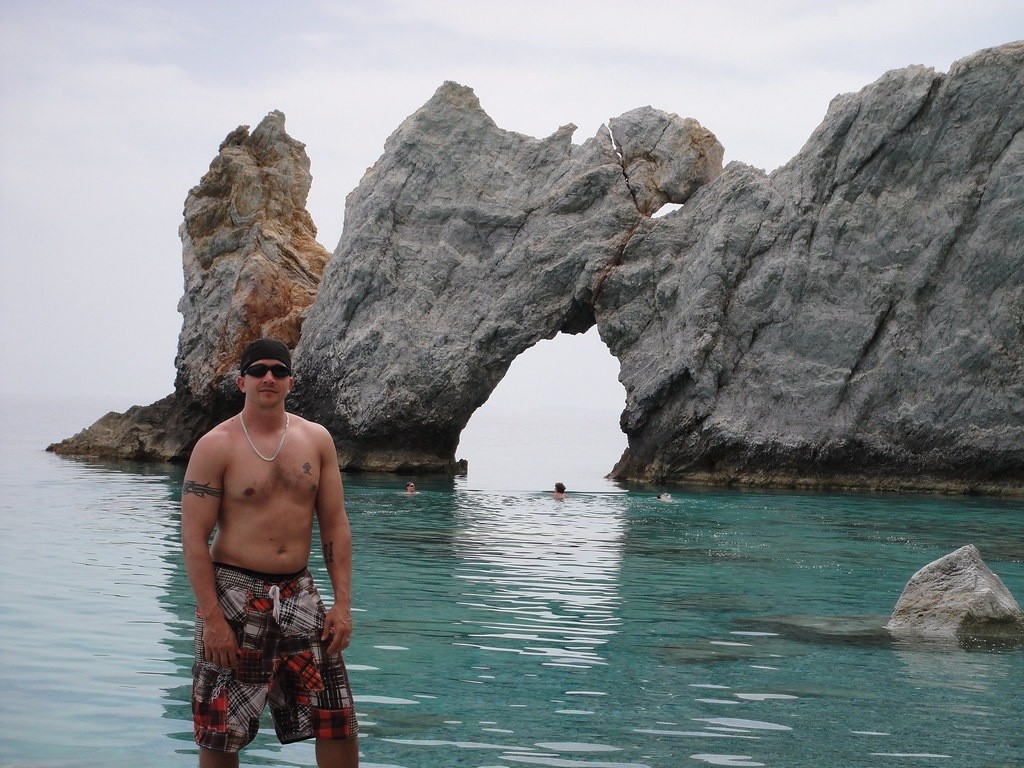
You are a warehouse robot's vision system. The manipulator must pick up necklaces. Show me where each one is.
[238,410,290,463]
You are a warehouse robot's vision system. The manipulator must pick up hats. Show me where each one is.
[240,338,291,375]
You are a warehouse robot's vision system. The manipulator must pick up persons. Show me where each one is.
[405,482,415,492]
[656,493,672,499]
[553,483,567,493]
[180,340,362,768]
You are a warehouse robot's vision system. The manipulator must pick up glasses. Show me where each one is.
[242,365,292,378]
